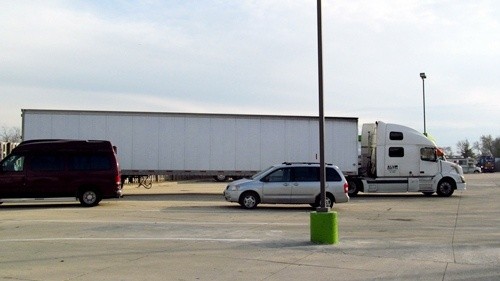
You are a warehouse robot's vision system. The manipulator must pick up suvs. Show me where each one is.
[224,162,350,209]
[0,140,123,207]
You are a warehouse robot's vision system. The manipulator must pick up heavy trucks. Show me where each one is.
[21,108,464,196]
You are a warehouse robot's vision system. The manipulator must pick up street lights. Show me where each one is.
[419,73,428,137]
